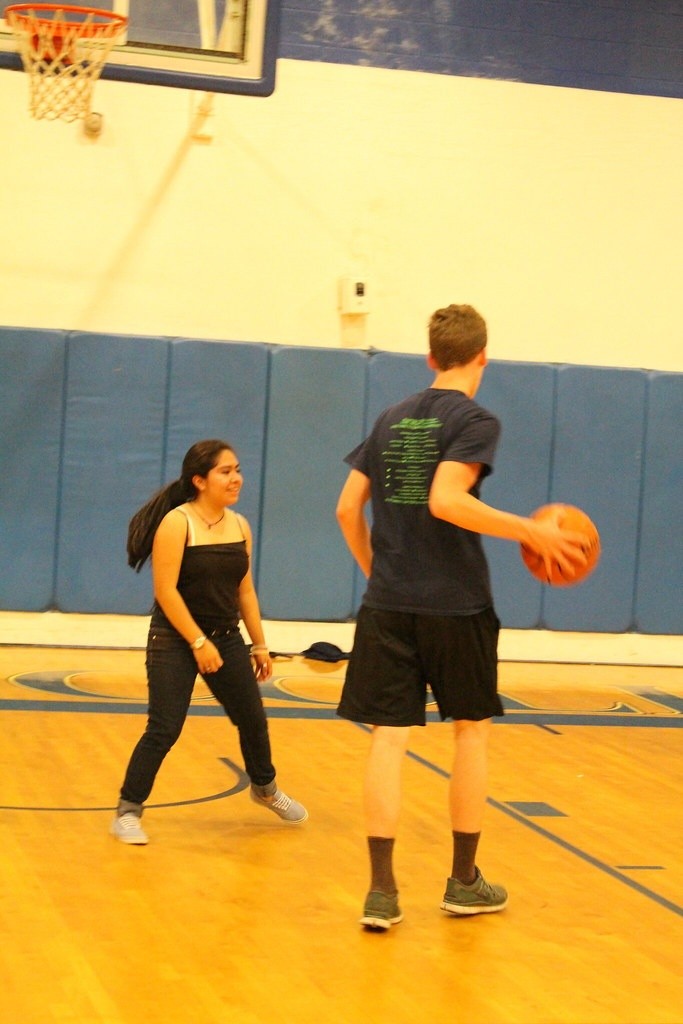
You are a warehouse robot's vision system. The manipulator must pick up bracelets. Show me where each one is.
[206,667,211,672]
[248,645,269,655]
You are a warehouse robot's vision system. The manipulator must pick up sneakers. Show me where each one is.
[359,890,403,929]
[110,812,149,844]
[250,788,309,824]
[439,865,508,914]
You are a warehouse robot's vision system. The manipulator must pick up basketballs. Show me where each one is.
[521,503,602,588]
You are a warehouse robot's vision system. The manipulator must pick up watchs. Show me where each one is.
[188,636,208,651]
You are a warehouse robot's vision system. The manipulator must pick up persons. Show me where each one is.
[336,304,591,929]
[105,439,308,844]
